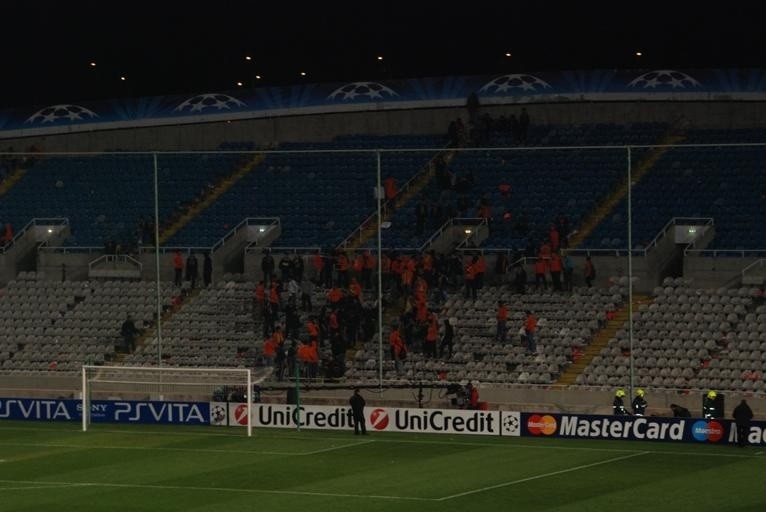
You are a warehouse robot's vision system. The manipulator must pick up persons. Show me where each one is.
[19,144,42,166]
[582,256,595,287]
[203,253,213,286]
[106,215,147,260]
[214,384,260,403]
[183,251,196,283]
[612,390,629,414]
[2,146,18,175]
[631,390,648,415]
[253,250,572,384]
[670,403,692,418]
[465,382,480,408]
[2,223,17,245]
[386,93,568,256]
[173,248,184,287]
[704,390,722,419]
[121,315,137,355]
[348,389,367,435]
[733,399,754,445]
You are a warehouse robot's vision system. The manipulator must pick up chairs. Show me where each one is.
[1,125,765,402]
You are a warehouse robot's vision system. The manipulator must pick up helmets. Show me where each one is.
[635,390,646,396]
[615,390,626,398]
[707,391,717,400]
